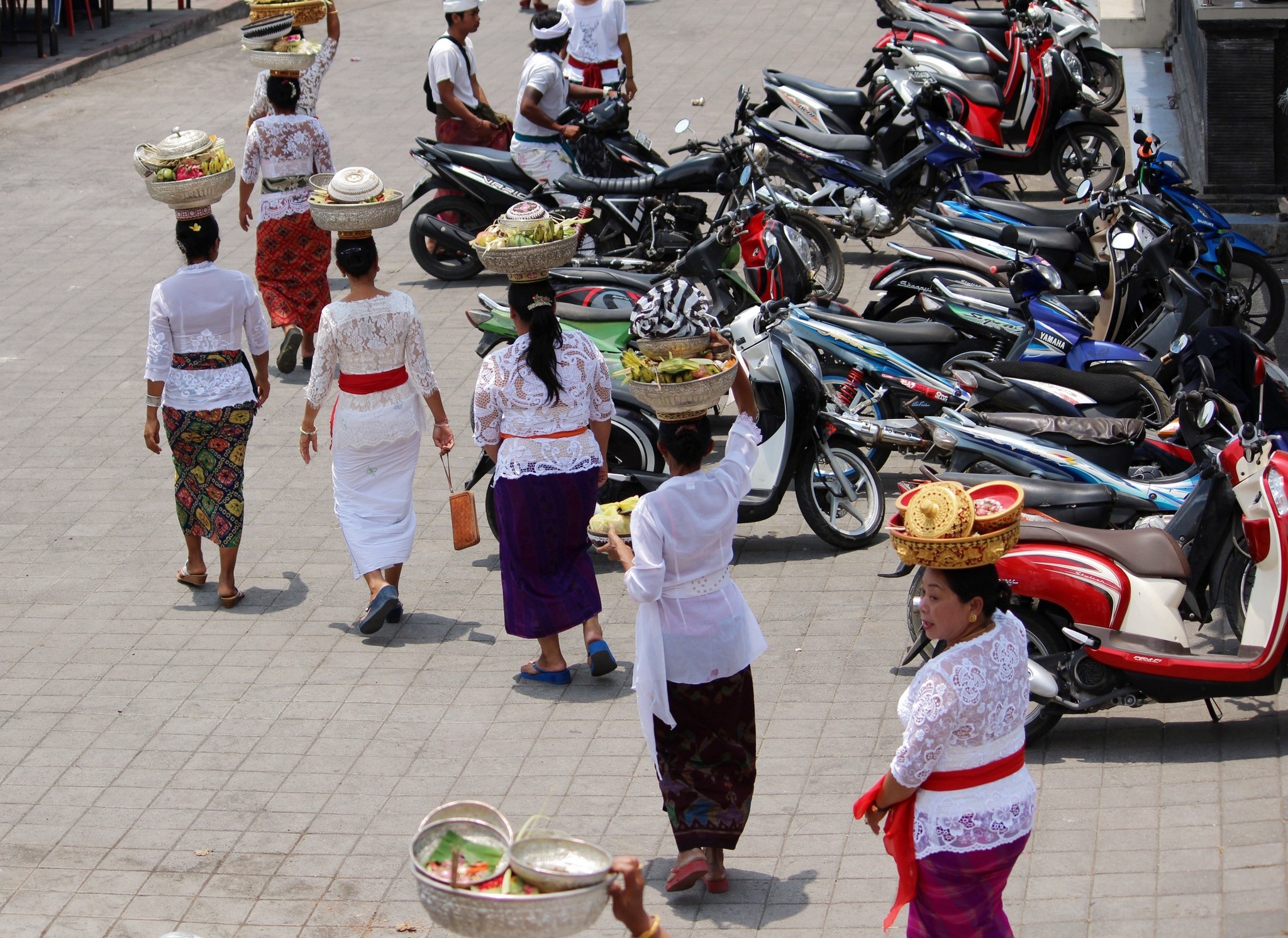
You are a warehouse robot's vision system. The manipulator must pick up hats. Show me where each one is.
[443,0,479,13]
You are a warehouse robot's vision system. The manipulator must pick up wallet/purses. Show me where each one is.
[439,451,480,551]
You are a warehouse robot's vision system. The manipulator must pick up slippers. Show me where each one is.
[386,601,403,623]
[276,328,303,374]
[217,586,245,608]
[521,661,571,685]
[176,560,208,586]
[359,585,399,635]
[665,860,709,892]
[302,357,312,370]
[701,876,728,894]
[430,248,468,261]
[587,640,617,677]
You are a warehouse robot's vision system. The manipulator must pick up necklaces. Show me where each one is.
[944,621,995,650]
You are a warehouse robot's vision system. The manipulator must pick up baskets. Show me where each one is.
[249,0,327,27]
[144,163,236,210]
[309,173,336,191]
[327,166,384,204]
[307,188,405,232]
[889,509,1021,569]
[904,480,975,538]
[133,126,213,179]
[636,335,710,361]
[241,12,294,51]
[896,484,930,521]
[411,818,511,888]
[624,353,738,414]
[249,49,316,71]
[965,479,1025,533]
[508,837,614,892]
[497,201,550,235]
[410,845,619,938]
[417,800,515,846]
[474,226,579,274]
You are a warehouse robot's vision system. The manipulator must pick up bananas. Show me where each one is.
[533,217,564,244]
[202,147,234,176]
[619,347,694,384]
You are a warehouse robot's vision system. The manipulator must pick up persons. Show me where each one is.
[143,214,271,609]
[473,277,618,685]
[606,855,672,938]
[594,327,767,893]
[245,0,340,135]
[508,10,631,256]
[238,75,337,374]
[555,0,637,116]
[426,0,514,261]
[299,230,455,635]
[864,561,1037,938]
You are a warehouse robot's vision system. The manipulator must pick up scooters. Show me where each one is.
[400,0,1287,743]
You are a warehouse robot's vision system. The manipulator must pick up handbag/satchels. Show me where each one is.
[422,36,471,115]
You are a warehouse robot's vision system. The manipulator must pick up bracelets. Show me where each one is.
[246,122,250,131]
[603,85,613,99]
[631,915,661,938]
[626,76,634,78]
[473,118,483,130]
[434,423,449,427]
[299,425,318,436]
[326,10,338,14]
[871,799,893,812]
[434,417,448,423]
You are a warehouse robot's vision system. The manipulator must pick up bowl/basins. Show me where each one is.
[133,129,237,211]
[472,203,580,274]
[306,166,404,233]
[888,476,1024,570]
[408,801,617,937]
[241,1,327,71]
[622,331,737,416]
